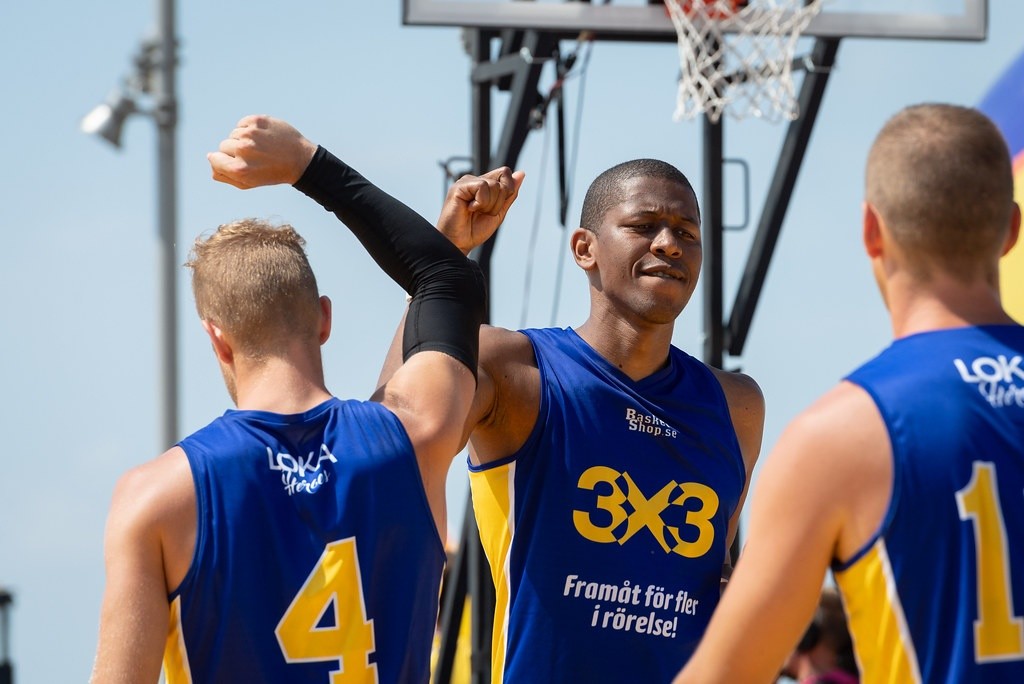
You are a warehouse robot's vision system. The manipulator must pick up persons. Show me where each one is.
[89,113,489,684]
[670,103,1022,684]
[377,160,765,684]
